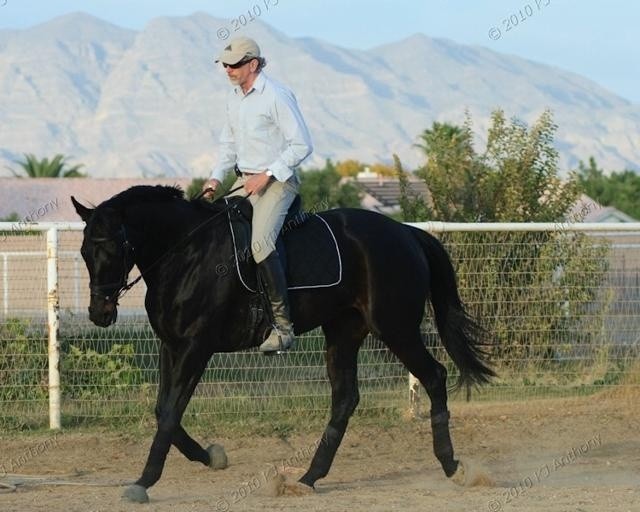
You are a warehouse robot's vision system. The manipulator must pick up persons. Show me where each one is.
[202,37,314,353]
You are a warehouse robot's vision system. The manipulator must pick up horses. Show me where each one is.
[70,181,502,504]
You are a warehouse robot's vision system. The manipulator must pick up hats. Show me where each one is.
[212,35,262,66]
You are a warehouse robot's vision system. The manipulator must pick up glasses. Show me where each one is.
[220,57,256,69]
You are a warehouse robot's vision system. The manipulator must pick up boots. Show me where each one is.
[256,249,296,353]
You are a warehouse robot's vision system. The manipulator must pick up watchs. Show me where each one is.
[264,168,276,181]
